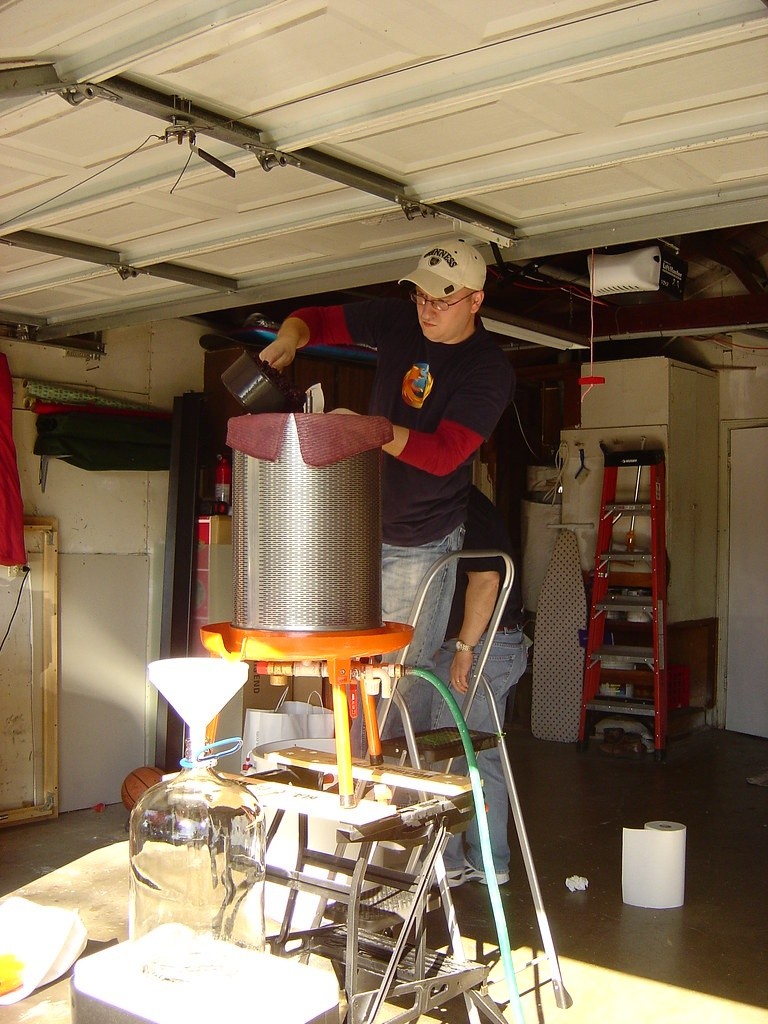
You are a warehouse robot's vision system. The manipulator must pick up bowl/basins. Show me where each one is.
[221,350,289,414]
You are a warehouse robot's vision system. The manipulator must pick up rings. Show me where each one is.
[456,680,460,684]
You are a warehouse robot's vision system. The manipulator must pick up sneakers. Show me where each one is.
[465,856,511,886]
[414,859,464,887]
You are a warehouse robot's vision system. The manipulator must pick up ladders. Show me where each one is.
[578,440,670,767]
[300,548,573,1024]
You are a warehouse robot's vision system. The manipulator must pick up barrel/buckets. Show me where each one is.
[229,411,384,633]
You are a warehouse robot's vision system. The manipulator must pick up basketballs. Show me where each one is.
[120,765,168,813]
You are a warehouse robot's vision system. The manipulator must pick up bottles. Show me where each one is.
[126,736,267,952]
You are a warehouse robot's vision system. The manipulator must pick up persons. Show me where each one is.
[421,485,527,884]
[260,239,514,807]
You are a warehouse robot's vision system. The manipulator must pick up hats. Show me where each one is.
[398,239,487,298]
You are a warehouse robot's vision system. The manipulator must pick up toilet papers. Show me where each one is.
[621,820,687,909]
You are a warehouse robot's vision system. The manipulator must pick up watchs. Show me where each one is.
[456,640,476,652]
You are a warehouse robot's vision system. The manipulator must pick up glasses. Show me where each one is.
[409,287,479,311]
